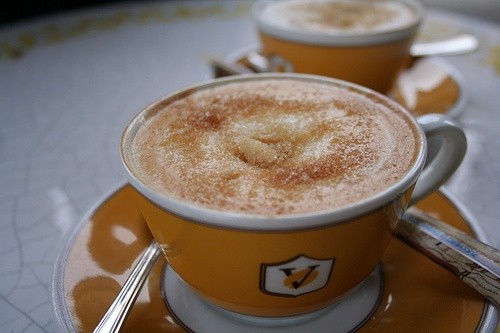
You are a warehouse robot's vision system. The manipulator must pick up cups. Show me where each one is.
[253,0,422,98]
[119,71,468,319]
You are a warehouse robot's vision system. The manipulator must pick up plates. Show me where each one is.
[212,41,470,127]
[51,170,494,333]
